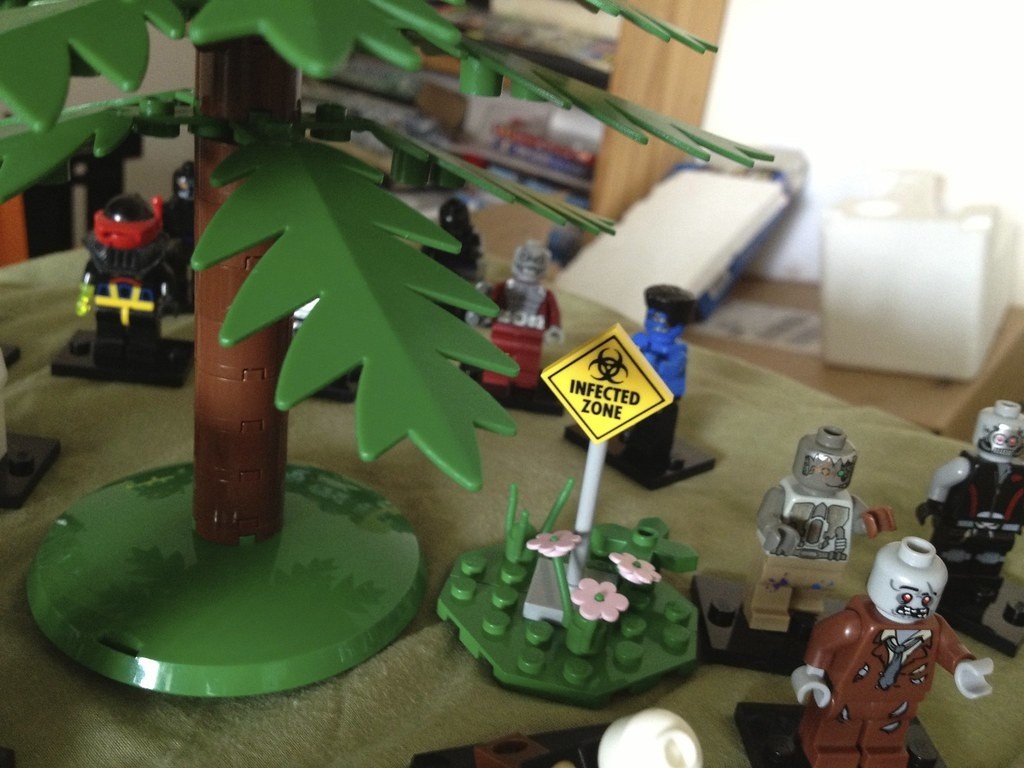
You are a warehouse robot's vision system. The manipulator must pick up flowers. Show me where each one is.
[607,551,662,585]
[570,578,630,622]
[526,528,582,558]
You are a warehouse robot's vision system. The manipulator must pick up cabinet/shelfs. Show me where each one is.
[818,206,1014,387]
[554,144,807,327]
[291,0,729,243]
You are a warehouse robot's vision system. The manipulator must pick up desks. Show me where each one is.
[0,241,1024,768]
